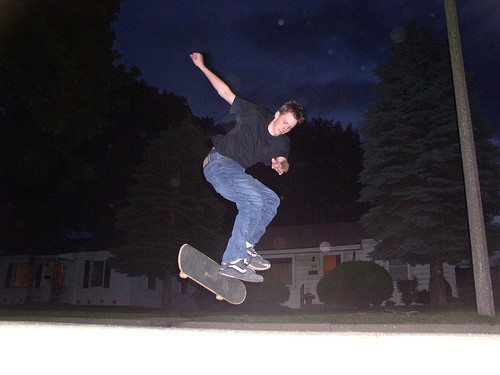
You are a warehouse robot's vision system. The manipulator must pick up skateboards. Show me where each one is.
[176,242,248,306]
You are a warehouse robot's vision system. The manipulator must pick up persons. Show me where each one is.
[189,52,304,283]
